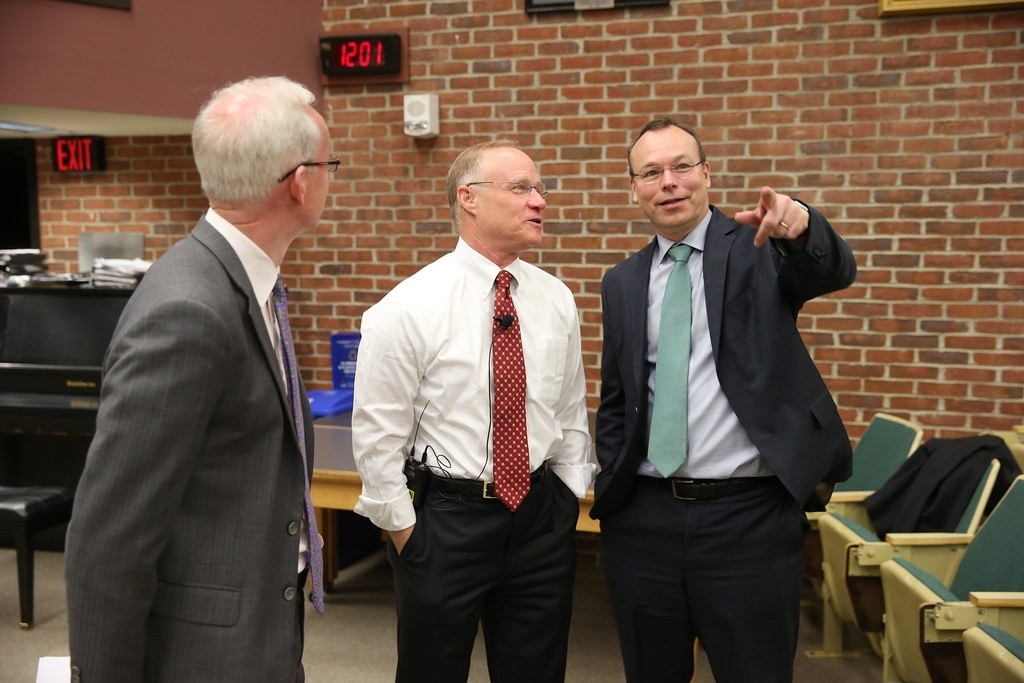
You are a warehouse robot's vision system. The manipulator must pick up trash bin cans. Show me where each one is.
[305,390,353,419]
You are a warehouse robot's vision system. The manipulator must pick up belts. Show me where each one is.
[429,459,549,498]
[621,474,778,500]
[297,566,310,589]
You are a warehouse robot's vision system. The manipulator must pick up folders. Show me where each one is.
[91,265,144,288]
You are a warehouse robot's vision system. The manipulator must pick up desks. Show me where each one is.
[311,401,601,598]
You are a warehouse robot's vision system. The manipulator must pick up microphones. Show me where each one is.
[500,314,514,329]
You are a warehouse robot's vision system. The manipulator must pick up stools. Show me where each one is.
[0,471,76,629]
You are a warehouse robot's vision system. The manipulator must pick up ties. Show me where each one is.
[272,277,324,616]
[647,245,693,478]
[492,270,530,513]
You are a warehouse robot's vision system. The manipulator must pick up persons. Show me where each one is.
[590,120,857,683]
[352,138,597,683]
[64,75,342,683]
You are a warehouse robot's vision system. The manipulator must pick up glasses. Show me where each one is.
[633,160,704,184]
[467,181,547,200]
[278,154,340,183]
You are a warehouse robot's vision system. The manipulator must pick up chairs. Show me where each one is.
[805,412,1024,683]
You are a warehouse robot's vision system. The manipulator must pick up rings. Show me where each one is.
[779,221,789,229]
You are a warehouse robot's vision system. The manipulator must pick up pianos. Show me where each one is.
[0,284,141,552]
